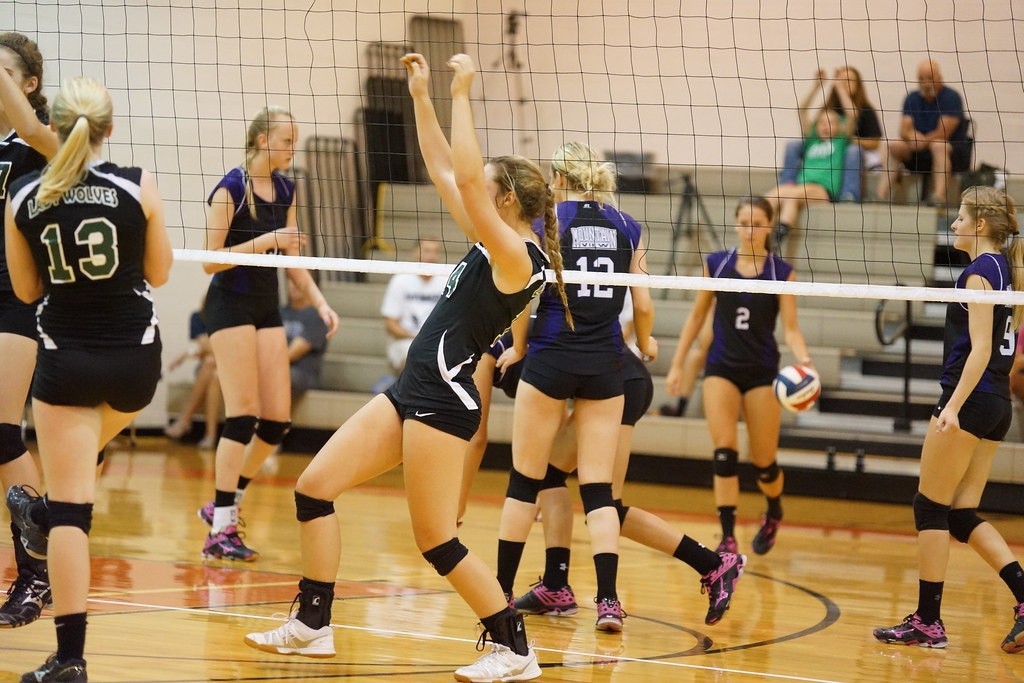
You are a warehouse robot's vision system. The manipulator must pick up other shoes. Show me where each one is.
[165,416,192,438]
[197,437,217,448]
[655,403,674,415]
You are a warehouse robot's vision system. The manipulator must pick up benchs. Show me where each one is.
[160,166,1023,494]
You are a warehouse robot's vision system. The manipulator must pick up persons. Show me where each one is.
[5,76,175,683]
[167,63,1024,656]
[0,31,66,630]
[244,54,577,683]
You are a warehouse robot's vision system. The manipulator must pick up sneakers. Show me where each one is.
[20,651,88,683]
[454,640,543,683]
[873,609,949,648]
[514,575,579,617]
[593,596,627,632]
[715,536,739,553]
[752,505,784,554]
[1000,603,1024,653]
[243,608,336,658]
[201,525,255,561]
[0,568,53,629]
[700,552,747,626]
[6,483,48,560]
[197,500,246,529]
[507,590,514,609]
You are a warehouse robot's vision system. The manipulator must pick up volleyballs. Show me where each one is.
[773,364,821,412]
